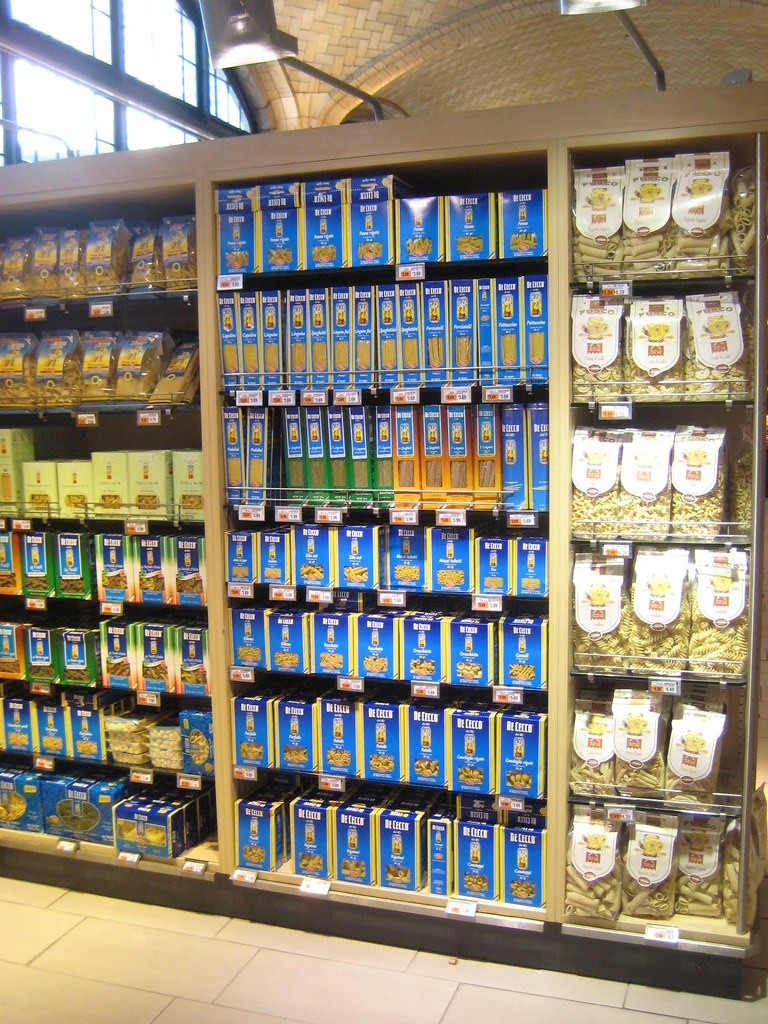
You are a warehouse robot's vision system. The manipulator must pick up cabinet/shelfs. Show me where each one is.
[0,82,768,1001]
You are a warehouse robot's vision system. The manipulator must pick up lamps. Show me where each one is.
[198,0,384,122]
[561,0,666,92]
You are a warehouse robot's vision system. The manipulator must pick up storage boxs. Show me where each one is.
[0,761,141,846]
[171,448,205,521]
[168,531,212,696]
[134,620,177,693]
[127,791,201,850]
[0,531,141,760]
[143,713,184,769]
[106,748,150,765]
[90,450,130,519]
[105,738,148,754]
[103,715,173,742]
[179,709,214,777]
[132,533,174,606]
[0,425,94,517]
[112,799,186,860]
[213,173,549,910]
[143,782,214,842]
[103,705,170,732]
[128,450,174,520]
